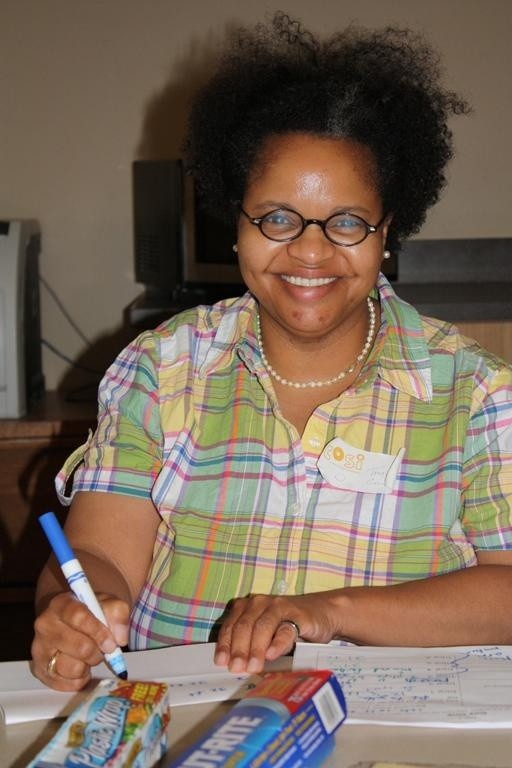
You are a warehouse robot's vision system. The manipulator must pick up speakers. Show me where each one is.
[131,156,183,289]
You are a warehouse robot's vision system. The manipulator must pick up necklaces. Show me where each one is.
[256,298,376,390]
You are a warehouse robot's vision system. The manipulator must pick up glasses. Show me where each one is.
[240,207,390,247]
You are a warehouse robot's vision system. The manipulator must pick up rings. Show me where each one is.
[46,650,58,677]
[281,618,300,638]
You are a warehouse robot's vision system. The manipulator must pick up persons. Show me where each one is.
[28,9,511,692]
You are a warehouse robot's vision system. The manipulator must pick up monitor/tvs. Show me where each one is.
[181,168,247,286]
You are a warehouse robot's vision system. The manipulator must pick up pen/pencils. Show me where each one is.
[38,509,128,682]
